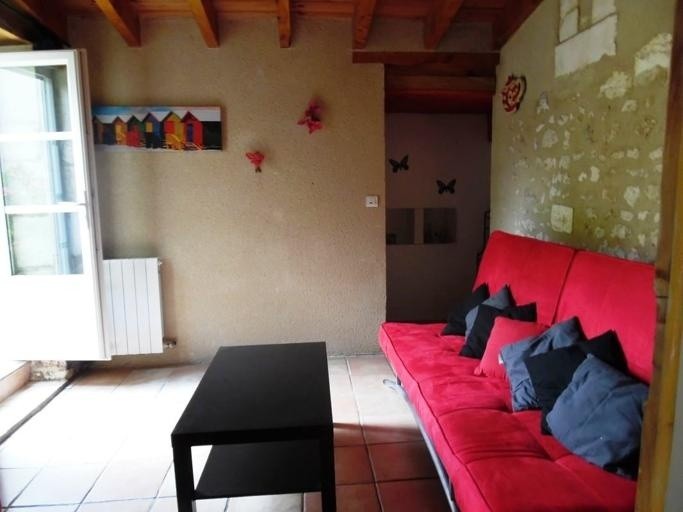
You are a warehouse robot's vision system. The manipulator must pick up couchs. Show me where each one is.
[380,231,656,508]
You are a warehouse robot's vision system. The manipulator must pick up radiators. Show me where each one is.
[102,257,176,357]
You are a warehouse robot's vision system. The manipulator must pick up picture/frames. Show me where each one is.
[91,105,223,151]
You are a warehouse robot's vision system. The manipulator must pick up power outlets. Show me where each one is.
[365,195,378,207]
